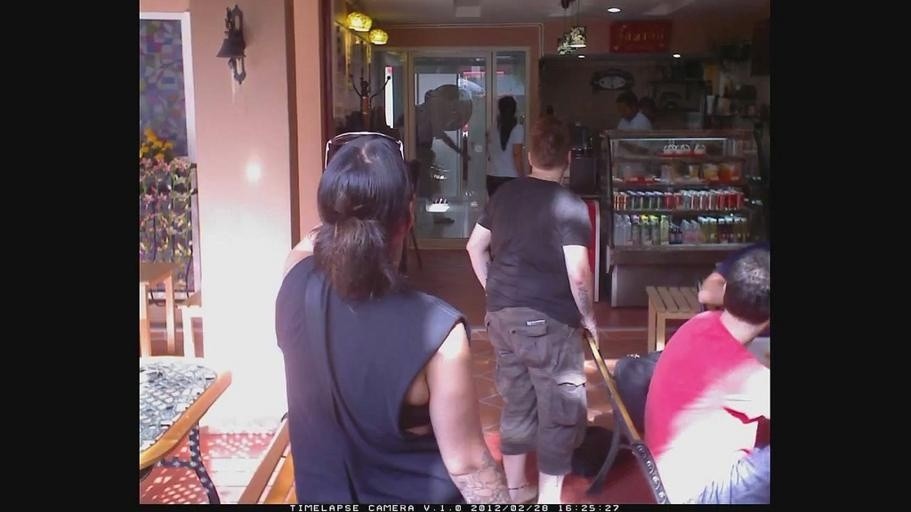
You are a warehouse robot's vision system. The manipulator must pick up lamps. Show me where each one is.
[346,11,389,46]
[555,1,589,63]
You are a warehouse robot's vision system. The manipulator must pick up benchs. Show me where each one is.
[585,330,672,504]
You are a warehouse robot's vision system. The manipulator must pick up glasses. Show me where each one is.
[324,130,405,170]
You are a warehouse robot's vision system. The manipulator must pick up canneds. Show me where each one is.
[613,188,745,210]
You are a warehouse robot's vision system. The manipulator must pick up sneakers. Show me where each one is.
[434,217,455,223]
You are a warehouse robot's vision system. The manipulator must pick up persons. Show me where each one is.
[273,130,513,505]
[640,97,675,153]
[481,96,525,197]
[613,92,656,177]
[394,87,472,227]
[464,127,604,506]
[696,237,770,338]
[141,96,179,139]
[642,249,771,505]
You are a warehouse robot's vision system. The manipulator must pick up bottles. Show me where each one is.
[613,212,749,246]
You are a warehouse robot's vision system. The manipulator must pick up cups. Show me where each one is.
[705,93,716,115]
[662,161,737,184]
